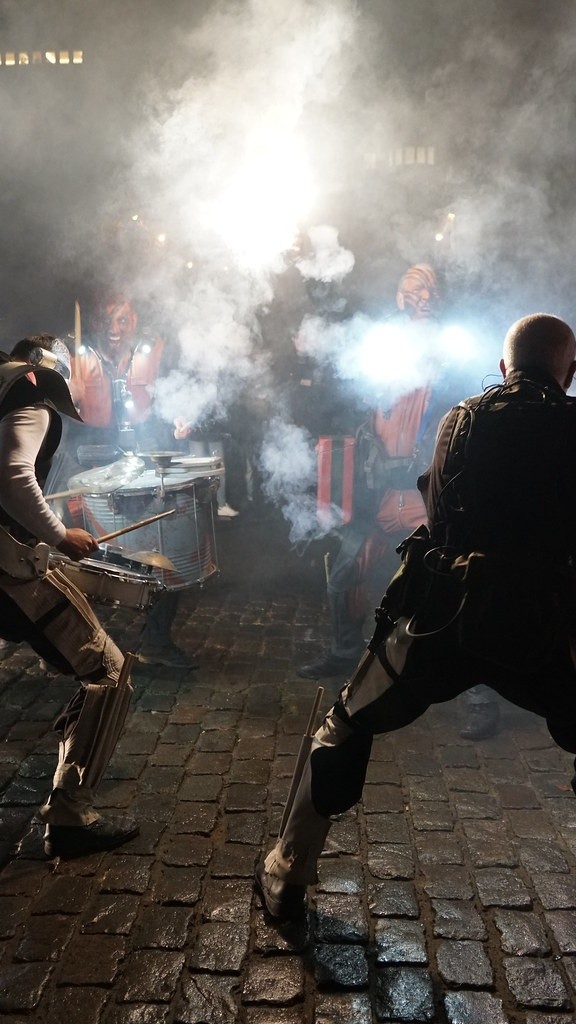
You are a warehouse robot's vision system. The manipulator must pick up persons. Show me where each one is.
[251,311,576,930]
[67,262,475,680]
[0,333,144,858]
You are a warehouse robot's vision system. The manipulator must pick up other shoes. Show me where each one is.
[140,636,200,670]
[459,702,500,741]
[44,815,140,859]
[299,649,361,679]
[255,861,307,918]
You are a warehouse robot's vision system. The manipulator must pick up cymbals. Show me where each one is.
[66,456,146,495]
[121,546,183,575]
[134,449,186,458]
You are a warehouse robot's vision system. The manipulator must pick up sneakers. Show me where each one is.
[217,503,239,516]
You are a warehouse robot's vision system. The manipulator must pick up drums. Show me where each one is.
[39,551,160,676]
[81,466,219,594]
